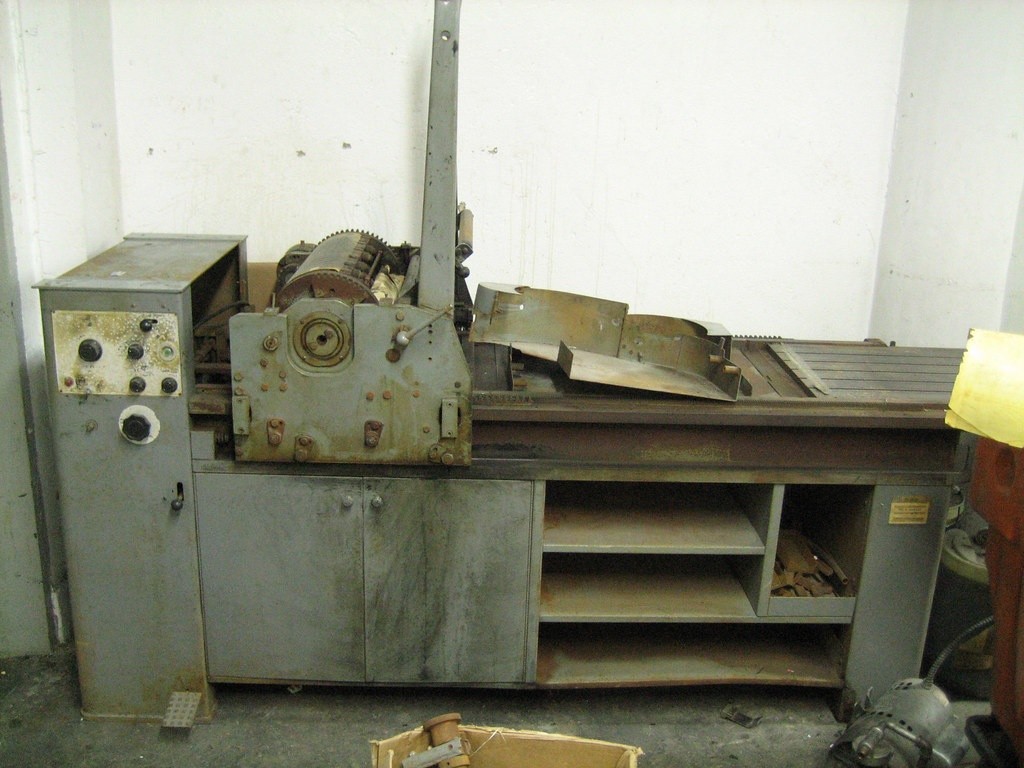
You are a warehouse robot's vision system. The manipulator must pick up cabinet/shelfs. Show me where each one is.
[190,458,950,722]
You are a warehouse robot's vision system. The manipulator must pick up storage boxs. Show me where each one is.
[369,724,642,768]
